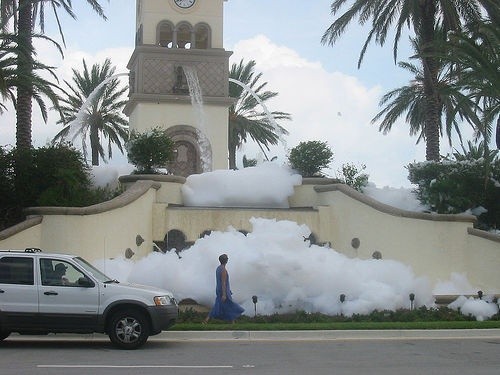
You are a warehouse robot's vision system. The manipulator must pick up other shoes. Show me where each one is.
[202,321,208,330]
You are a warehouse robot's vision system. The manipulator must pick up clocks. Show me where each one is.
[169,0,199,14]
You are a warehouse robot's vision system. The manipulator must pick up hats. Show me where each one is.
[55,263,68,272]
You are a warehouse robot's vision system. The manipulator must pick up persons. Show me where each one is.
[201,253,245,326]
[49,262,68,285]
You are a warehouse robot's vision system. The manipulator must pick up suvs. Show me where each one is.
[1,247,180,350]
